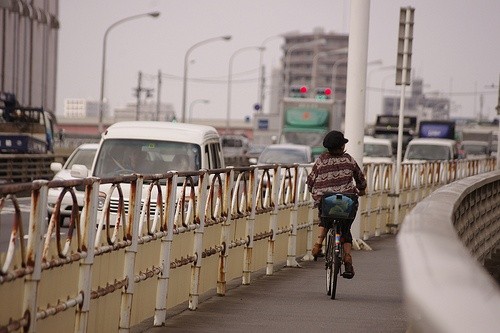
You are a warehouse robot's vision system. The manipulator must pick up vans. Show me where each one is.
[79,121,226,252]
[403,139,498,162]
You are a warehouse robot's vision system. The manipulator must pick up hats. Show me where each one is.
[323,131,348,147]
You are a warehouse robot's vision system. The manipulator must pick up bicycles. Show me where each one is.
[318,193,365,299]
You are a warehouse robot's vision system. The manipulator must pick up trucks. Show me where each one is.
[373,114,457,154]
[271,83,344,163]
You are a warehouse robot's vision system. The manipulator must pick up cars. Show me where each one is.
[220,135,311,197]
[47,143,100,226]
[363,136,393,178]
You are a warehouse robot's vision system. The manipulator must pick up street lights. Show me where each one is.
[98,11,159,138]
[182,36,232,124]
[227,31,294,131]
[276,39,405,149]
[409,84,495,139]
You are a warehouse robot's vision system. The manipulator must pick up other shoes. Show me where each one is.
[344,252,354,280]
[312,242,322,262]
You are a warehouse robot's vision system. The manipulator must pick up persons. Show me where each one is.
[307,131,367,278]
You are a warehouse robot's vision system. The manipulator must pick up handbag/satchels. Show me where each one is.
[326,194,354,211]
